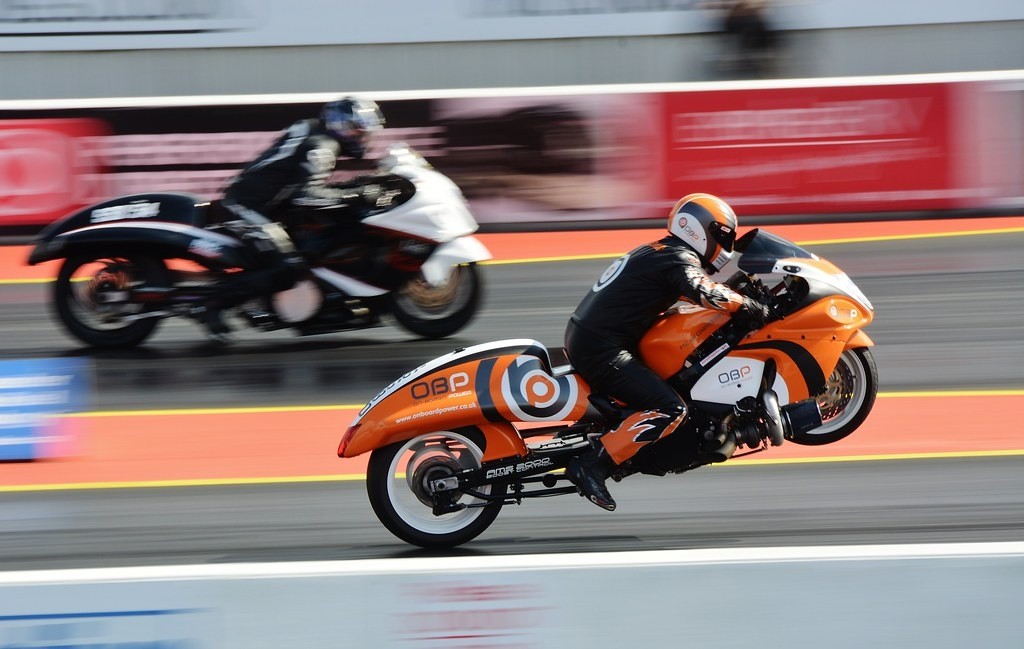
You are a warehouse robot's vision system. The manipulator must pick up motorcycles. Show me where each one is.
[336,225,880,552]
[26,140,495,349]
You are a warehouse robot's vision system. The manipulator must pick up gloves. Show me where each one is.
[731,295,775,329]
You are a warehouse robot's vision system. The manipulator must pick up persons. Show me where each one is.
[565,192,768,510]
[211,95,386,337]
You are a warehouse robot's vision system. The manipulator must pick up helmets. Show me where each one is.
[322,96,388,157]
[666,193,738,275]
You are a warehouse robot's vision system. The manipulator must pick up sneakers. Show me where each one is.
[564,461,617,511]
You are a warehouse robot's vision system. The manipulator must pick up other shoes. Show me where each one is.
[186,293,239,343]
